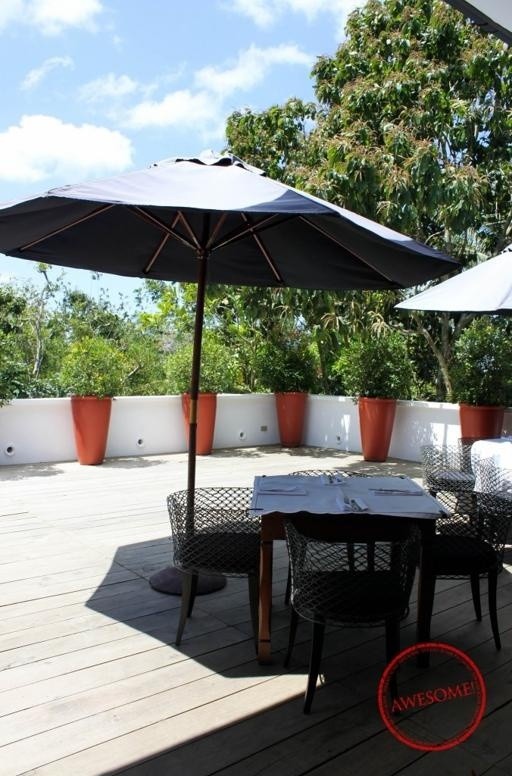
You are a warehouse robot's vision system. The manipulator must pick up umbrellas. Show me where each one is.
[0,154,465,538]
[393,243,512,312]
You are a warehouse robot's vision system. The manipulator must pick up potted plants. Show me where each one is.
[169,341,232,455]
[59,335,129,465]
[254,332,320,448]
[446,314,512,445]
[329,326,415,462]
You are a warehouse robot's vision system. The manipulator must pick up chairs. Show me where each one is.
[166,437,512,715]
